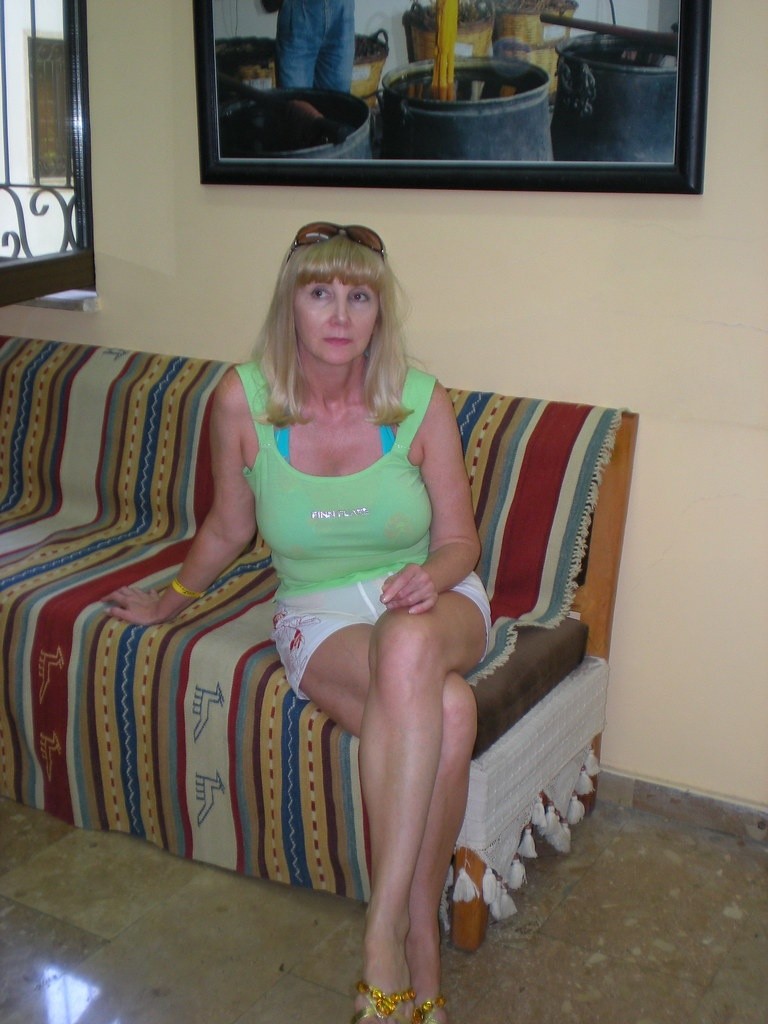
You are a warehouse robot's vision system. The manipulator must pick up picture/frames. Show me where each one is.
[193,0,712,194]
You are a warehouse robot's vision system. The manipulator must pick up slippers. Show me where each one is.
[352,979,417,1024]
[413,993,448,1024]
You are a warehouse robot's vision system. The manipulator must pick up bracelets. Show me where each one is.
[172,577,204,598]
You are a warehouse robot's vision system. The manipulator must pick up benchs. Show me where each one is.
[0,337,640,954]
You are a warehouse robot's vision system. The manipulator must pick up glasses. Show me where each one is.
[287,222,385,263]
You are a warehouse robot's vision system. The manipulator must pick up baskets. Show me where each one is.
[349,29,389,98]
[215,36,276,90]
[403,0,496,63]
[497,0,579,95]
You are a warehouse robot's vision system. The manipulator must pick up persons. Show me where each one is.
[276,0,355,93]
[102,222,496,1024]
